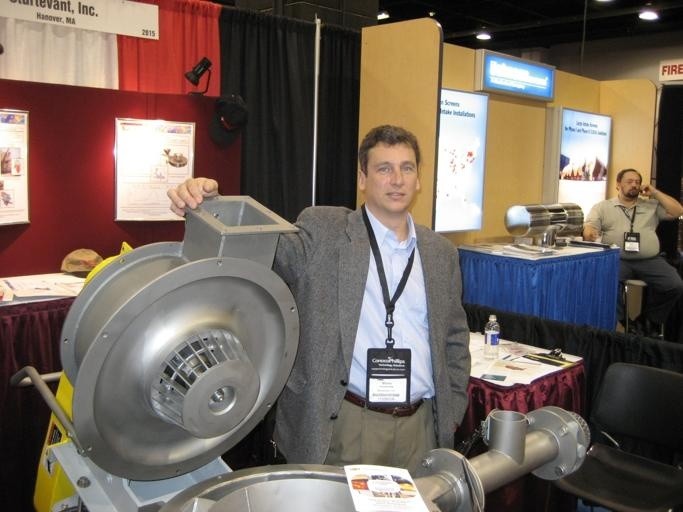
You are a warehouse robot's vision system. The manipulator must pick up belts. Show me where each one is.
[344,391,423,416]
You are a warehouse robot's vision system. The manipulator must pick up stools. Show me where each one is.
[622,279,666,341]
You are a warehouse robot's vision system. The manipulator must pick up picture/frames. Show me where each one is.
[113,117,196,223]
[0,108,30,226]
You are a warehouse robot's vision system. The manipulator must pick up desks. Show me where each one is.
[455,334,586,512]
[456,239,620,332]
[1,273,86,512]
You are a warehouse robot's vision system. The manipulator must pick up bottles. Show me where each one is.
[484,315,500,358]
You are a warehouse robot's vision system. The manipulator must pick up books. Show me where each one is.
[503,244,560,258]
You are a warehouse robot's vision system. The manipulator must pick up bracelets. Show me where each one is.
[652,189,659,199]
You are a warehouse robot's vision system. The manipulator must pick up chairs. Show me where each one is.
[541,362,682,512]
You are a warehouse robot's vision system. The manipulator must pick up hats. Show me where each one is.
[208,94,249,145]
[61,248,103,274]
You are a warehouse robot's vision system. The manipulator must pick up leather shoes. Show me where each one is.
[620,314,659,339]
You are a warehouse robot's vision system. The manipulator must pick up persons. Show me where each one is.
[584,169,683,337]
[166,125,471,478]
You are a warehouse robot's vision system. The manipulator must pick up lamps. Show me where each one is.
[184,57,212,98]
[638,0,661,22]
[376,7,392,20]
[475,22,492,41]
